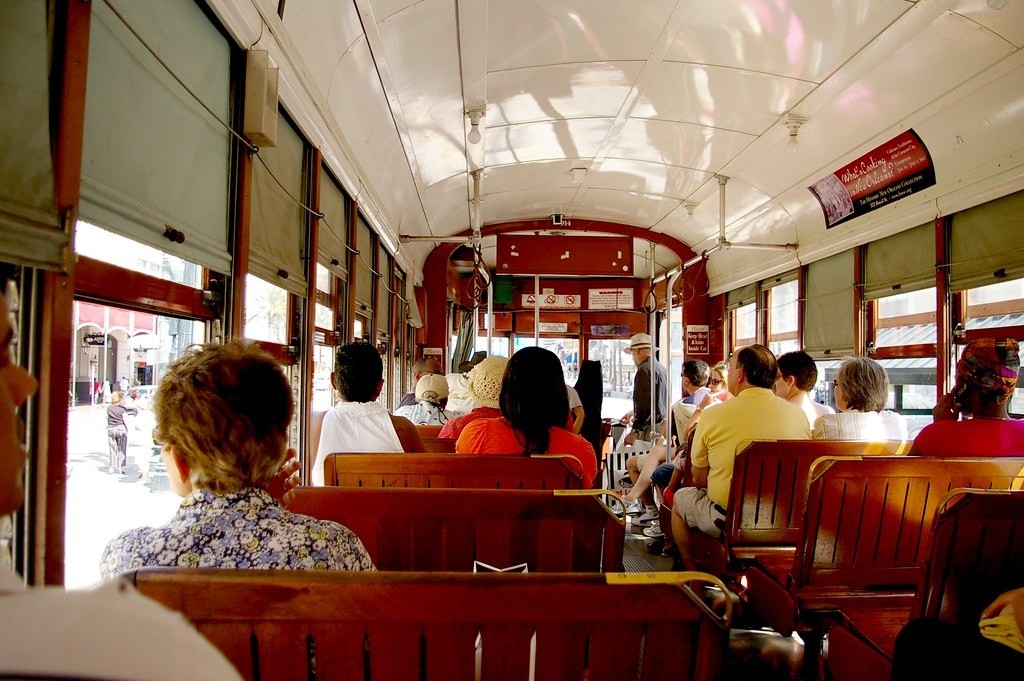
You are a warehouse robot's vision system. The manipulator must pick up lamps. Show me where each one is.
[80,338,89,355]
[137,345,143,357]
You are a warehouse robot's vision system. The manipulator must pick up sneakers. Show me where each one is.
[642,520,665,537]
[608,494,638,514]
[638,508,660,520]
[617,494,642,512]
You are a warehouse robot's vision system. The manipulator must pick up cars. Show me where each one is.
[885,392,933,439]
[602,378,611,397]
[121,386,157,414]
[314,373,331,391]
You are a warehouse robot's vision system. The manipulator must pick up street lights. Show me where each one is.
[90,359,98,407]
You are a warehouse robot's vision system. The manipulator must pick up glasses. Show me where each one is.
[833,379,839,390]
[708,376,725,386]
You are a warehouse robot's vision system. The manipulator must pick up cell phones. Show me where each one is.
[952,382,973,405]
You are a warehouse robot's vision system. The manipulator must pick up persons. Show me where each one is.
[607,333,834,614]
[565,383,585,434]
[100,336,380,681]
[308,343,426,484]
[813,356,908,441]
[455,346,597,492]
[0,267,244,681]
[906,336,1024,458]
[890,587,1024,681]
[392,349,510,443]
[89,375,156,485]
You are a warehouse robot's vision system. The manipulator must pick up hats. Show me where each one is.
[458,351,487,372]
[624,332,663,354]
[468,355,511,409]
[415,373,450,402]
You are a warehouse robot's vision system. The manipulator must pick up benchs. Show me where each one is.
[120,426,1024,681]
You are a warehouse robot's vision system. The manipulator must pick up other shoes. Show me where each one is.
[647,538,676,553]
